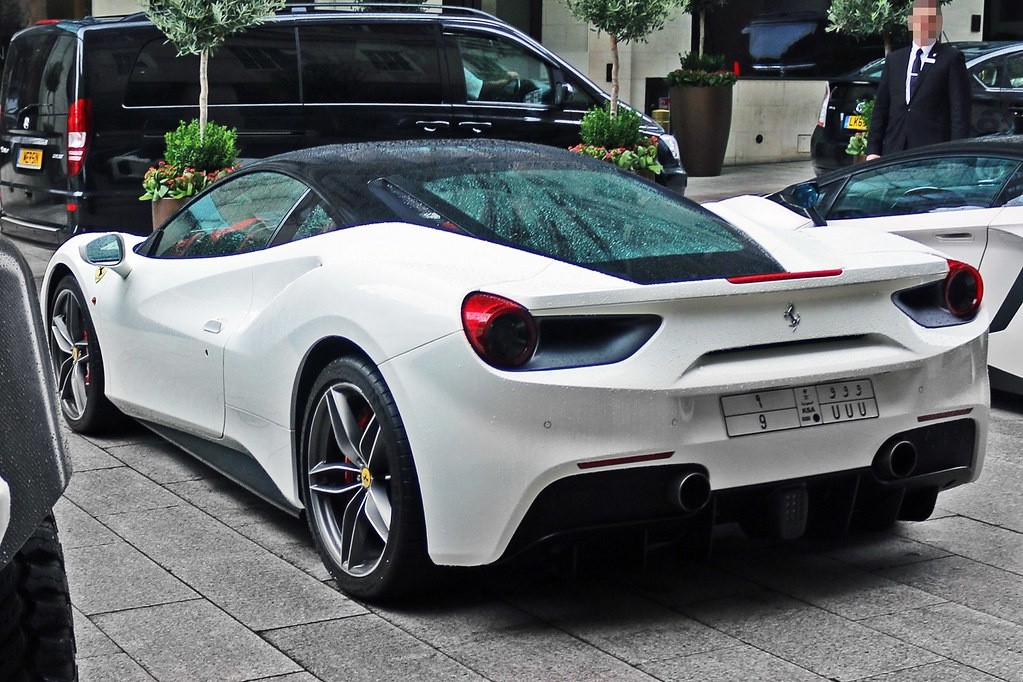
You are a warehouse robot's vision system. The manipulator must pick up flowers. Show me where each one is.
[138,118,244,201]
[568,98,666,175]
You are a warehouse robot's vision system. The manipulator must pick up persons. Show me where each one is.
[462,63,519,101]
[866,0,972,161]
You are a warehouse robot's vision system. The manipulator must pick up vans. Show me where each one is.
[0,0,690,253]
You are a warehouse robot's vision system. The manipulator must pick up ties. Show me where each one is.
[910,48,924,104]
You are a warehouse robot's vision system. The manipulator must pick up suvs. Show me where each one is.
[808,38,1023,184]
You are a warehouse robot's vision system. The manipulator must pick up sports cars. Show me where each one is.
[759,132,1023,401]
[36,136,992,608]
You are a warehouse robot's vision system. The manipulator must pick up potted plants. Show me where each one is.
[666,50,737,177]
[147,0,285,234]
